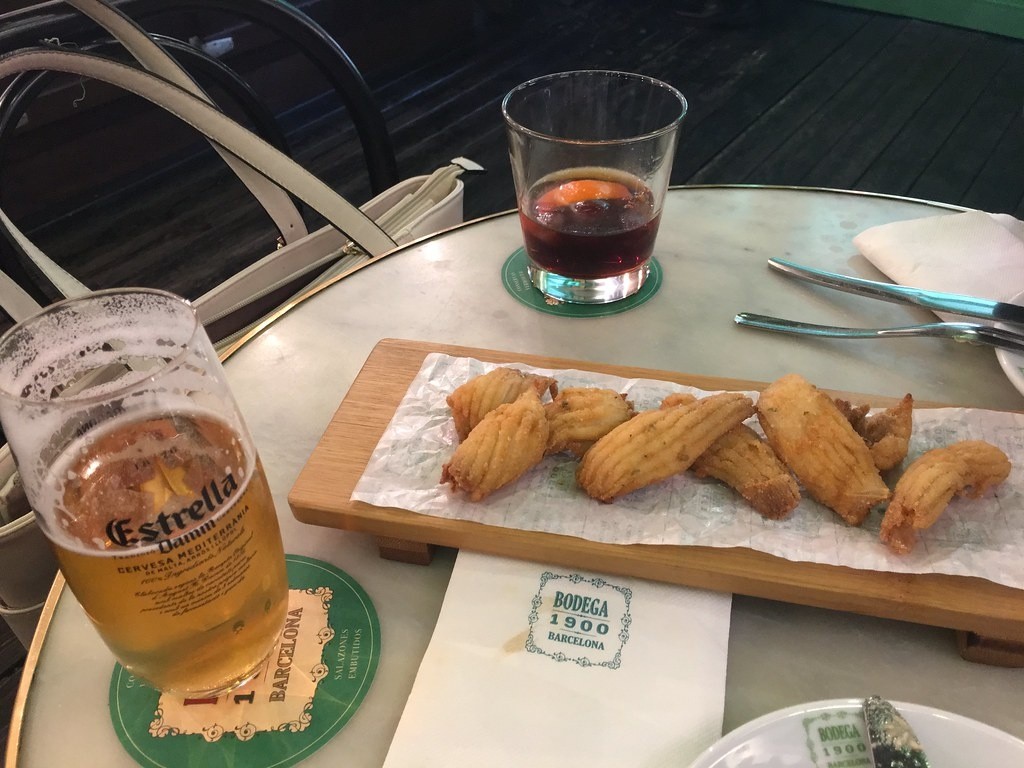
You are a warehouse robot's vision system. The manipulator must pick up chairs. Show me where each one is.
[0,2,403,307]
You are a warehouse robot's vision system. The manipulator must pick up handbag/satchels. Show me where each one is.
[1,0,487,656]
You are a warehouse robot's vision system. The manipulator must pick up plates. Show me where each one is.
[992,289,1024,398]
[687,697,1024,768]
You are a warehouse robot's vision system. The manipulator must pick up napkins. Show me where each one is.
[380,548,732,768]
[849,211,1023,348]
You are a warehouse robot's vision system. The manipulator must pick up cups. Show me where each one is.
[0,287,290,700]
[501,68,689,306]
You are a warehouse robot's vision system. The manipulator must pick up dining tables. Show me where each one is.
[0,179,1024,767]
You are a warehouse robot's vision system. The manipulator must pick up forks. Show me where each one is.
[733,312,1024,354]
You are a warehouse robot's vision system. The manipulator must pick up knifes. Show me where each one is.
[766,256,1024,325]
[862,695,933,768]
